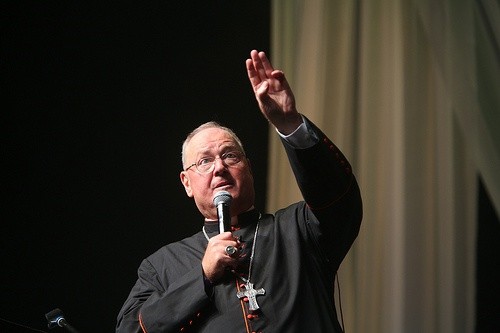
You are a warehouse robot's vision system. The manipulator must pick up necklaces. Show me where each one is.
[201,211,266,310]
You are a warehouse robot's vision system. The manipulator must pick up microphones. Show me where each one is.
[213,191,234,275]
[45,309,72,331]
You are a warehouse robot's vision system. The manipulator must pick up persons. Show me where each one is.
[117,49,363,333]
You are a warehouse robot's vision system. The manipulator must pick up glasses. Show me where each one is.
[186,151,244,173]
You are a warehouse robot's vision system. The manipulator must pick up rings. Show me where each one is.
[224,245,236,257]
